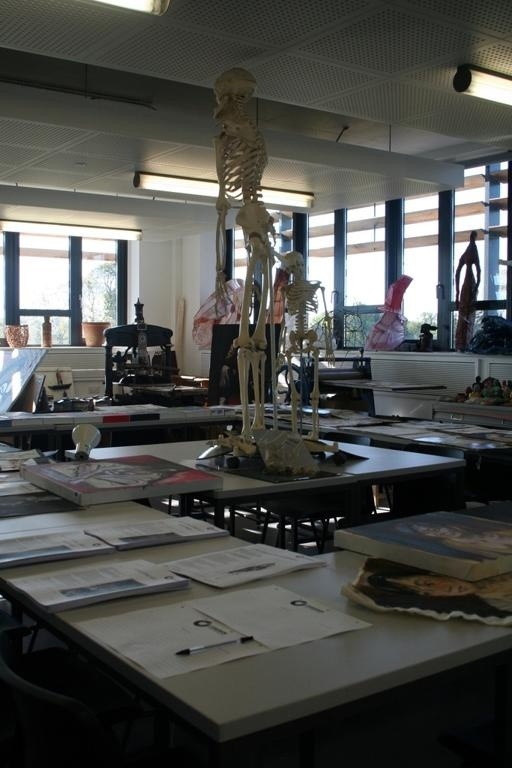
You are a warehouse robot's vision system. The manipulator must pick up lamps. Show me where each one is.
[452,66,512,106]
[92,0,171,17]
[133,169,316,210]
[0,218,141,242]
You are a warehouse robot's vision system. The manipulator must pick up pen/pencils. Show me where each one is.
[175,636,253,655]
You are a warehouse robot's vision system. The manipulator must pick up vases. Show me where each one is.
[80,321,111,347]
[4,324,30,348]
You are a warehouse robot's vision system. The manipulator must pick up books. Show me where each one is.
[1,448,511,617]
